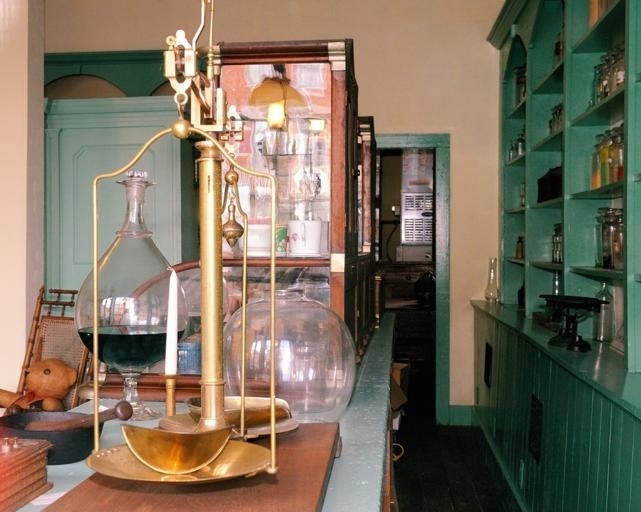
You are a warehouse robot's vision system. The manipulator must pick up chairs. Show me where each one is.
[18,286,93,410]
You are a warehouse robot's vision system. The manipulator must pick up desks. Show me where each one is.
[0,312,408,512]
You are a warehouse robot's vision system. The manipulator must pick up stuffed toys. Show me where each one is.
[0,358,78,417]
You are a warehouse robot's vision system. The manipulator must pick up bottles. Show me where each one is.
[514,63,527,105]
[518,279,525,307]
[547,270,562,320]
[592,36,627,108]
[508,123,527,163]
[552,222,563,264]
[553,26,566,70]
[484,257,502,303]
[550,103,564,133]
[517,236,524,259]
[595,204,624,268]
[592,121,624,190]
[594,280,615,342]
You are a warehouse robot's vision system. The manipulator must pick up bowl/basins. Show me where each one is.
[1,410,112,464]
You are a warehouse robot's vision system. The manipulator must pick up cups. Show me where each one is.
[287,221,324,258]
[179,341,202,377]
[275,225,288,257]
[243,224,273,258]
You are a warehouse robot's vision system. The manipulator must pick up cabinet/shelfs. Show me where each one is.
[44,96,199,322]
[471,0,640,511]
[76,38,380,401]
